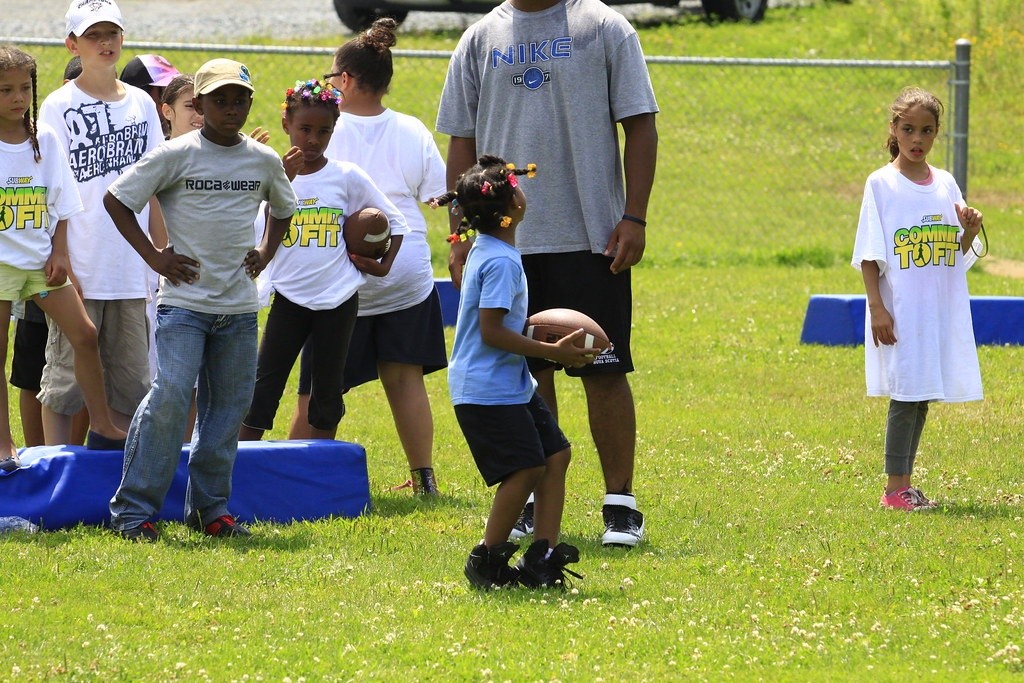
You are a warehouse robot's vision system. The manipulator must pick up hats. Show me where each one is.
[65,0,124,38]
[194,58,255,98]
[119,54,182,87]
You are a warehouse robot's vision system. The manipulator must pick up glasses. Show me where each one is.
[323,72,354,83]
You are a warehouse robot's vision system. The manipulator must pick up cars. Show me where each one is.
[330,0,770,34]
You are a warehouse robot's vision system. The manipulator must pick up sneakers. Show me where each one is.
[464,539,521,591]
[880,486,936,511]
[513,538,584,592]
[116,522,163,544]
[512,492,535,537]
[410,468,438,494]
[602,492,643,546]
[198,515,251,538]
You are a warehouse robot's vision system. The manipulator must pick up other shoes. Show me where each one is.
[0,455,21,471]
[86,429,127,450]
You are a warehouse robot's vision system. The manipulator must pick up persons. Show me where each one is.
[147,73,270,444]
[8,55,118,446]
[0,43,128,470]
[425,152,585,590]
[103,57,298,546]
[435,0,660,544]
[237,77,411,441]
[849,81,984,513]
[35,0,167,446]
[286,15,447,495]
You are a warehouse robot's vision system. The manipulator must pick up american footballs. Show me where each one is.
[524,308,612,363]
[344,207,392,260]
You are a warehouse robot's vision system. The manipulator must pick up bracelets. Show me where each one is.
[622,214,646,226]
